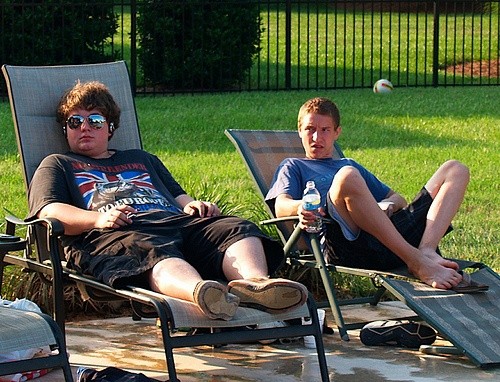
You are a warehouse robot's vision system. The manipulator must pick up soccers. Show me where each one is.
[372,78,393,94]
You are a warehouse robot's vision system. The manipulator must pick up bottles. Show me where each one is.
[303,181,322,233]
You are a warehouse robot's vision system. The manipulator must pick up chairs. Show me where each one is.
[4,60,330,382]
[0,234,74,381]
[224,128,500,368]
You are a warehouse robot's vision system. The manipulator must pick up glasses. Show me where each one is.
[65,113,107,130]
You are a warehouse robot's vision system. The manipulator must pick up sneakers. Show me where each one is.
[360,321,436,349]
[256,320,301,344]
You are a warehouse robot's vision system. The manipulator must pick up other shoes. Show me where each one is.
[227,276,309,315]
[192,280,240,321]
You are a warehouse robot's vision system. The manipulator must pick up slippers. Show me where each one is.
[447,270,489,292]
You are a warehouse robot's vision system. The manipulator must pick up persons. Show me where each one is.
[264,97,471,290]
[26,79,309,322]
